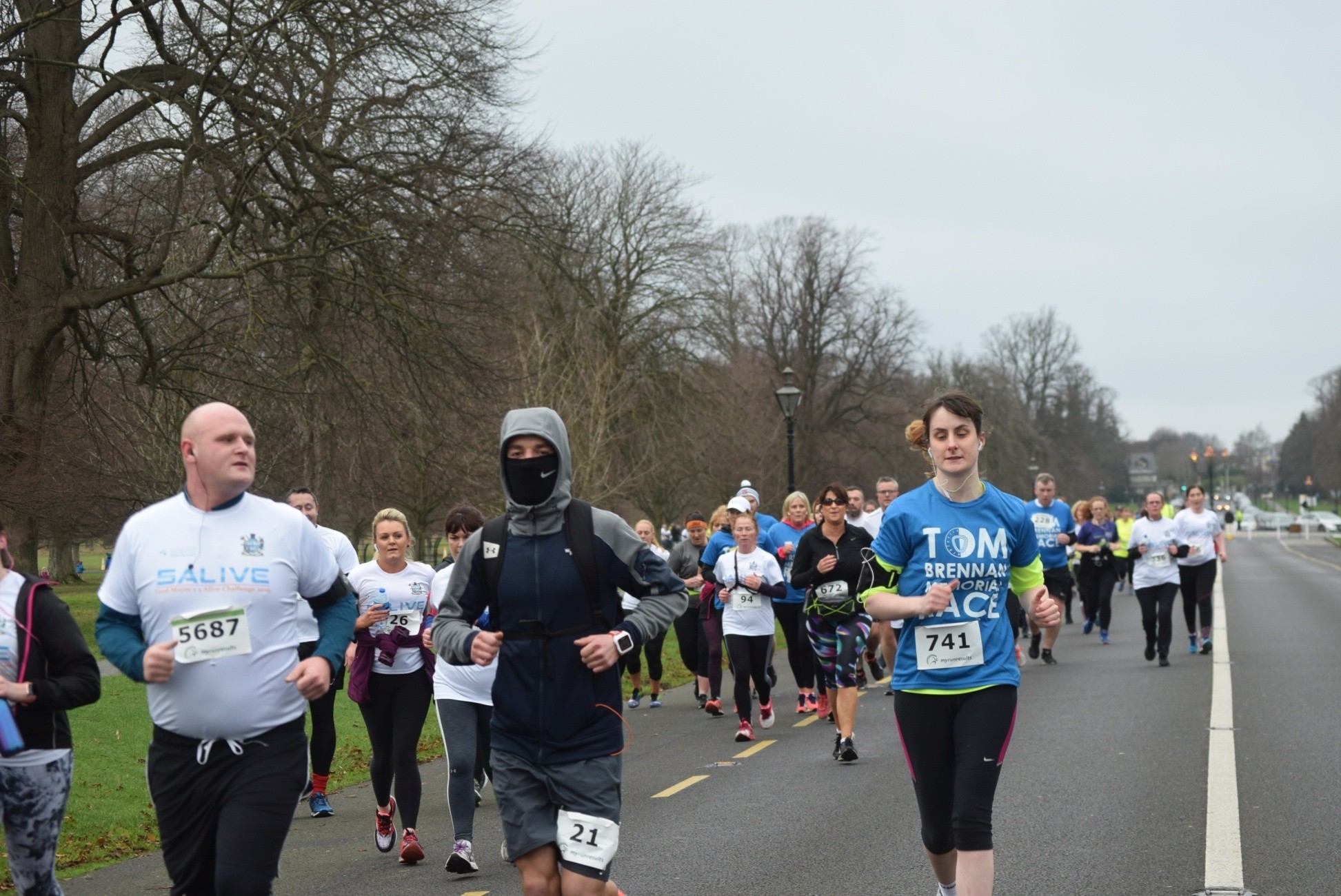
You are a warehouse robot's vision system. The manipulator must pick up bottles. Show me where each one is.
[1140,535,1151,560]
[373,588,390,629]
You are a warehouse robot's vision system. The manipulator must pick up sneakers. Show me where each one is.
[373,794,398,853]
[445,839,480,874]
[883,684,895,696]
[300,779,313,801]
[306,792,334,818]
[649,693,663,707]
[500,839,515,863]
[817,692,835,722]
[856,668,868,688]
[705,696,725,716]
[397,827,425,865]
[795,691,819,713]
[735,719,755,741]
[865,652,884,681]
[832,732,859,762]
[628,688,644,708]
[759,695,775,729]
[694,678,707,709]
[1011,580,1215,668]
[472,769,489,808]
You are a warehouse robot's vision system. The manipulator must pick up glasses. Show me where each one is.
[823,498,846,506]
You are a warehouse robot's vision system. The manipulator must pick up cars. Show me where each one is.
[1213,486,1341,532]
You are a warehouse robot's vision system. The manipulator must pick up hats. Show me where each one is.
[728,496,751,513]
[735,486,760,507]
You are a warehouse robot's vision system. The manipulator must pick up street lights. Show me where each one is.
[1305,475,1316,512]
[773,367,802,495]
[1188,443,1232,512]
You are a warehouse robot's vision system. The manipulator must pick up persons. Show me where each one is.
[1005,580,1032,668]
[697,505,739,717]
[1127,490,1191,668]
[422,504,513,875]
[346,507,439,865]
[843,476,900,540]
[280,486,361,818]
[428,406,691,896]
[0,522,102,896]
[700,496,778,689]
[1172,484,1229,656]
[734,485,779,532]
[764,490,819,714]
[1067,490,1243,604]
[39,551,112,580]
[1024,473,1078,665]
[802,496,839,722]
[621,520,671,709]
[790,482,875,762]
[94,402,359,896]
[666,511,713,709]
[1074,496,1122,644]
[859,390,1062,896]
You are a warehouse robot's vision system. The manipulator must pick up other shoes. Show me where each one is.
[878,654,887,668]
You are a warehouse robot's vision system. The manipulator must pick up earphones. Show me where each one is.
[188,448,193,458]
[978,441,983,452]
[928,447,933,459]
[374,543,378,553]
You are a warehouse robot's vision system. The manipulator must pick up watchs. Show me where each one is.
[20,680,37,708]
[607,629,635,656]
[329,671,336,686]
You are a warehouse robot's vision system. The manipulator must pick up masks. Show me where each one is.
[505,453,558,506]
